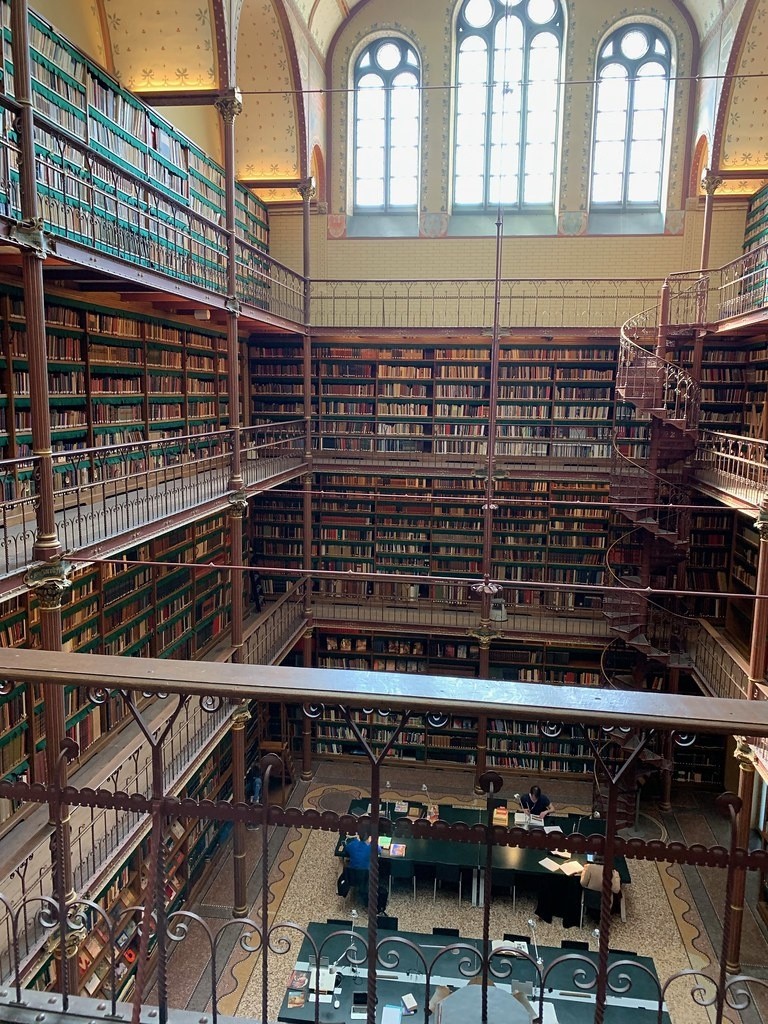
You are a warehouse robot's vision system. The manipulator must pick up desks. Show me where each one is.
[478,809,631,923]
[277,919,673,1024]
[333,799,481,907]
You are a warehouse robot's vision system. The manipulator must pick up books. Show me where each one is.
[731,182,768,317]
[14,682,278,1001]
[491,939,531,958]
[0,0,279,319]
[0,271,768,520]
[283,959,416,1024]
[292,628,720,783]
[235,484,767,622]
[0,491,239,848]
[490,805,595,877]
[366,798,441,857]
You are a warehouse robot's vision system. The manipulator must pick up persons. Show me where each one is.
[518,785,556,819]
[580,851,621,910]
[337,828,381,874]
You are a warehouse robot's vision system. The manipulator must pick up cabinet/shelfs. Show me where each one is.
[313,627,669,783]
[1,499,258,842]
[249,471,760,662]
[1,283,250,527]
[248,306,768,489]
[0,0,271,309]
[22,697,265,1003]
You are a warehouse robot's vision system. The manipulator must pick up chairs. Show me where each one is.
[579,887,613,929]
[377,916,398,930]
[343,866,369,886]
